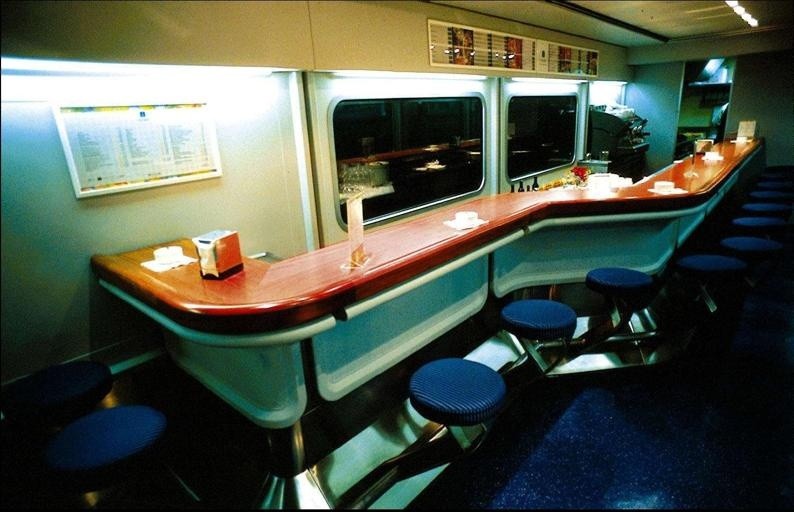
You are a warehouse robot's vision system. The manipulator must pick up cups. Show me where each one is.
[737,137,747,143]
[654,181,675,191]
[455,210,478,227]
[705,152,719,160]
[153,246,184,266]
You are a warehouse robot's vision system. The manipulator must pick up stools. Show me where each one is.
[0,361,112,429]
[43,403,169,494]
[502,299,577,379]
[676,165,793,314]
[586,268,653,343]
[409,358,507,463]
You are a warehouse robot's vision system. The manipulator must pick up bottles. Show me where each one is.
[510,184,515,193]
[532,177,540,191]
[527,185,530,191]
[518,180,524,192]
